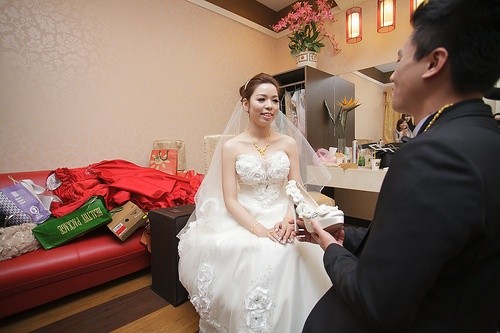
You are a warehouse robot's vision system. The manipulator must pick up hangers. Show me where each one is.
[283,84,306,97]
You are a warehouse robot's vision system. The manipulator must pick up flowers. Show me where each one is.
[269,0,342,54]
[334,96,358,116]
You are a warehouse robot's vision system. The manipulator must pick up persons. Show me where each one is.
[303,0,500,333]
[392,113,414,143]
[175,72,334,333]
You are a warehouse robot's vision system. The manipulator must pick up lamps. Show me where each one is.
[345,0,365,44]
[410,0,429,18]
[377,0,396,33]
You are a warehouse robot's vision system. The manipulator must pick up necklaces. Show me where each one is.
[246,129,272,155]
[424,104,453,133]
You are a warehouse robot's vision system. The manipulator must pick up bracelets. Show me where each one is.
[250,222,259,232]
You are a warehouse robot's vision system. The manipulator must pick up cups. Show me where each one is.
[370,159,381,170]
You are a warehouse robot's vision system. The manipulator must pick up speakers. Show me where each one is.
[148,203,195,307]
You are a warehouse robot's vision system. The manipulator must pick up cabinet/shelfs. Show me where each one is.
[271,66,357,149]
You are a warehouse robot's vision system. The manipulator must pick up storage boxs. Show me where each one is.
[107,203,144,242]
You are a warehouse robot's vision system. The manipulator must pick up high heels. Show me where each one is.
[285,180,345,234]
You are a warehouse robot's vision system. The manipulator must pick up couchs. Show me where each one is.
[0,160,204,323]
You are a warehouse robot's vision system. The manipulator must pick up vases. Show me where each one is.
[335,111,347,152]
[295,51,319,68]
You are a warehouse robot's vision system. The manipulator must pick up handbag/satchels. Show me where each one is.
[148,148,179,176]
[31,195,124,251]
